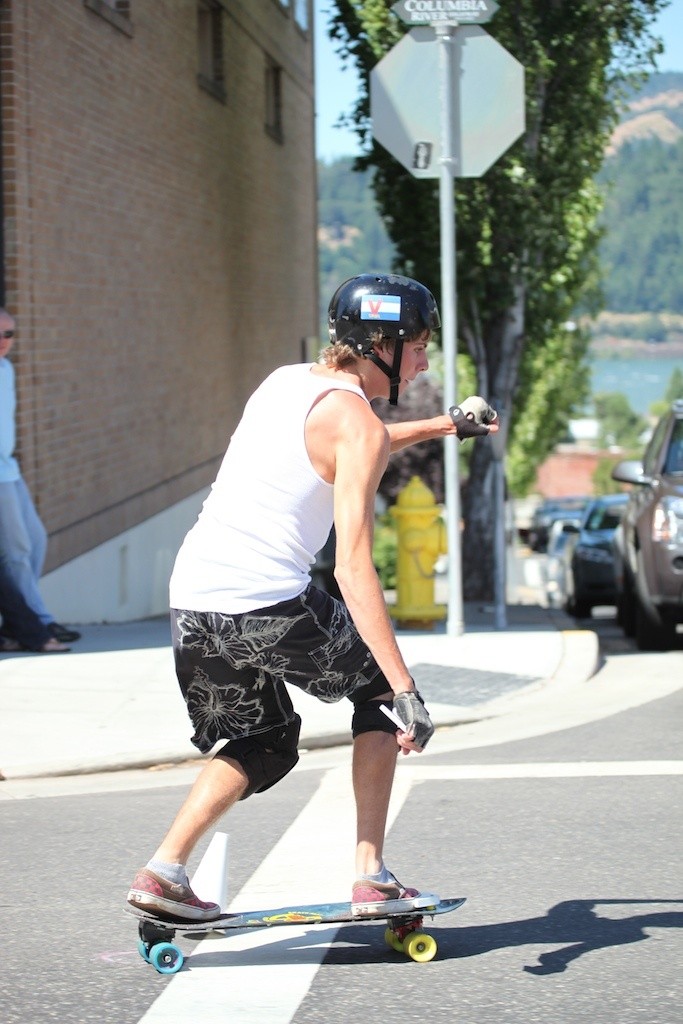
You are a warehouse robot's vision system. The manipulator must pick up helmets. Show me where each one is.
[327,273,443,355]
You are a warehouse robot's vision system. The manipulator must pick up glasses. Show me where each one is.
[0,330,14,339]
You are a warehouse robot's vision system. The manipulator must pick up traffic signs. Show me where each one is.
[389,0,501,25]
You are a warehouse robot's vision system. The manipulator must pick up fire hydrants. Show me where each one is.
[387,474,448,632]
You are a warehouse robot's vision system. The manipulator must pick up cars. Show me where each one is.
[558,492,634,620]
[608,399,683,650]
[514,497,596,611]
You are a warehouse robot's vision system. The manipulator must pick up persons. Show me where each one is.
[0,308,82,652]
[122,274,501,920]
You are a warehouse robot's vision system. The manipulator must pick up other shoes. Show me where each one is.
[0,637,22,651]
[46,623,80,642]
[45,637,72,652]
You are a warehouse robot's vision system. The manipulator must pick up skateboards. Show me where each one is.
[123,894,471,975]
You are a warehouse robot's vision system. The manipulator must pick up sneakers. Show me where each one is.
[126,865,220,921]
[350,873,442,917]
